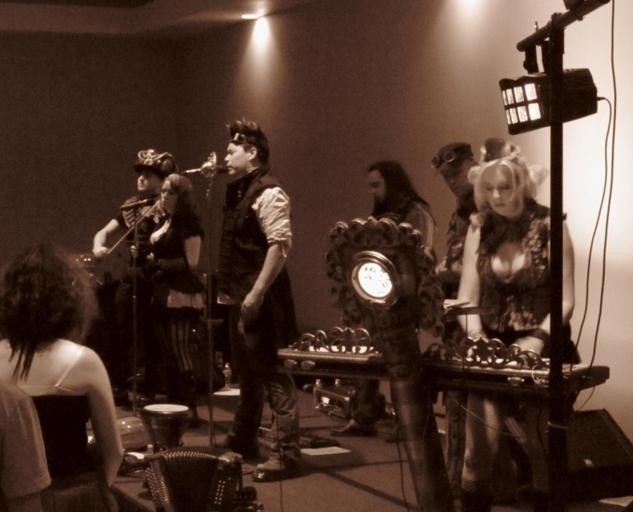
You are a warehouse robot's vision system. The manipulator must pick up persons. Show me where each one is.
[329,158,439,442]
[211,118,304,482]
[431,140,480,491]
[91,146,179,410]
[457,137,576,509]
[136,172,228,429]
[1,239,153,511]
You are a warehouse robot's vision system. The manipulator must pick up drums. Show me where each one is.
[142,403,189,487]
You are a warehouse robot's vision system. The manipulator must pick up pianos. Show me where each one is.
[276,330,611,396]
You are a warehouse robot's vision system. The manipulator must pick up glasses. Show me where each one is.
[227,132,252,145]
[429,151,457,169]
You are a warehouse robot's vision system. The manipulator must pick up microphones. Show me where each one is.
[183,165,227,176]
[120,198,155,210]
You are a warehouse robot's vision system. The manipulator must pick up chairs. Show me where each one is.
[0,380,269,512]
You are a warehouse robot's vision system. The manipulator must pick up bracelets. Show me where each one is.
[529,328,550,344]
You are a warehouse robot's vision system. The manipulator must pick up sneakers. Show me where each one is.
[249,446,306,484]
[207,434,262,460]
[330,418,379,438]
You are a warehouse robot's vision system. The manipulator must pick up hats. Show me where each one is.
[134,149,176,175]
[430,140,474,178]
